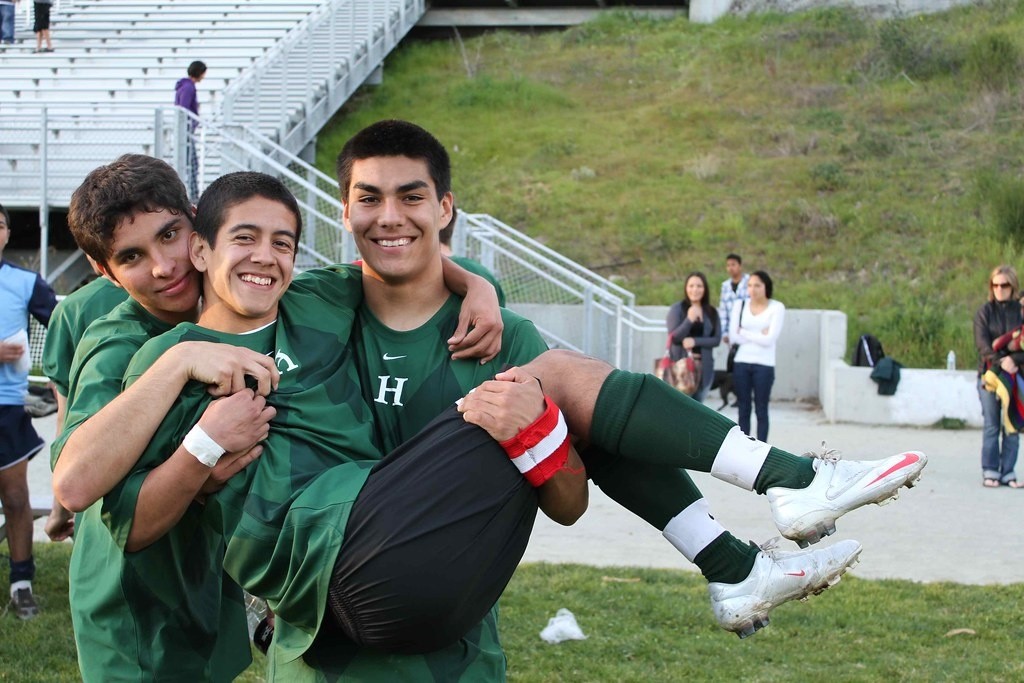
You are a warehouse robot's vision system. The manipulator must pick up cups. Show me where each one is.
[6,328,33,373]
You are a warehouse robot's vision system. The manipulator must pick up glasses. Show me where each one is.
[993,283,1010,289]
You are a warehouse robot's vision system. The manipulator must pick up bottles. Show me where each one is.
[946,350,955,370]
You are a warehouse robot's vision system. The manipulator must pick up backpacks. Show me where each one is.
[852,334,884,366]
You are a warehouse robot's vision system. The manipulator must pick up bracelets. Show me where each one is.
[499,395,585,488]
[182,424,225,468]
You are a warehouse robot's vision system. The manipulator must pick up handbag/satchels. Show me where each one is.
[726,343,738,371]
[654,332,701,396]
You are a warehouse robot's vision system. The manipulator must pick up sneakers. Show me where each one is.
[708,537,863,640]
[9,579,38,620]
[765,441,929,549]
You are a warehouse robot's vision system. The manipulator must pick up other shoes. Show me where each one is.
[982,477,998,487]
[1007,479,1023,488]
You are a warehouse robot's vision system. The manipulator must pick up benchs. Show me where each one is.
[0,0,325,206]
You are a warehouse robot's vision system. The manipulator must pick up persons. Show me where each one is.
[0,0,21,44]
[719,254,752,409]
[728,272,784,442]
[32,0,55,54]
[41,123,929,682]
[973,265,1024,489]
[174,61,209,200]
[666,272,722,405]
[1,202,103,615]
